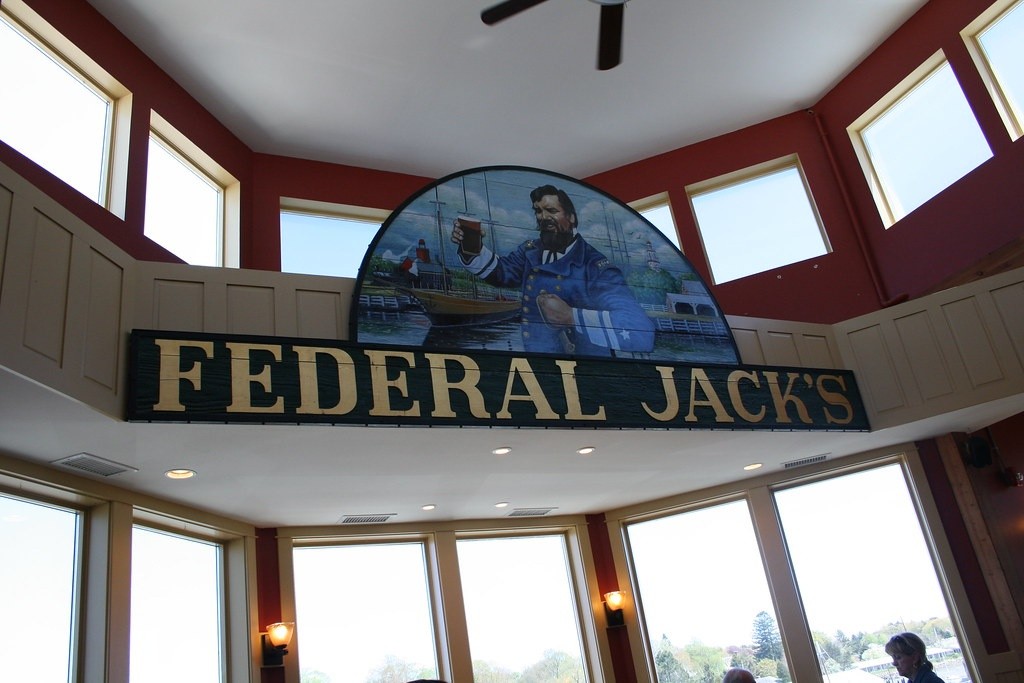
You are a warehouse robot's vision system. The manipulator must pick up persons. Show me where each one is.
[885,632,945,683]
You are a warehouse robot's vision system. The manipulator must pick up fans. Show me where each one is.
[481,0,630,72]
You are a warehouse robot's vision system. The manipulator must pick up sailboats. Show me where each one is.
[399,170,522,329]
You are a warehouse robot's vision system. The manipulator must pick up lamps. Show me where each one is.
[262,620,295,666]
[603,590,627,627]
